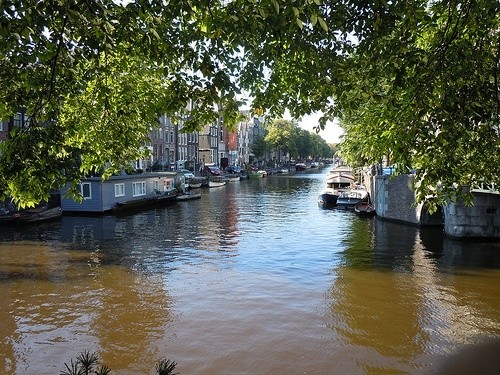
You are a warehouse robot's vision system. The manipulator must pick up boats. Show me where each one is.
[281,169,289,173]
[297,162,374,218]
[257,171,268,177]
[175,192,202,201]
[208,181,226,187]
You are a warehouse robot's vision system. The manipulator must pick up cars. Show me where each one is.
[180,168,194,179]
[248,164,259,171]
[225,164,241,175]
[200,165,221,176]
